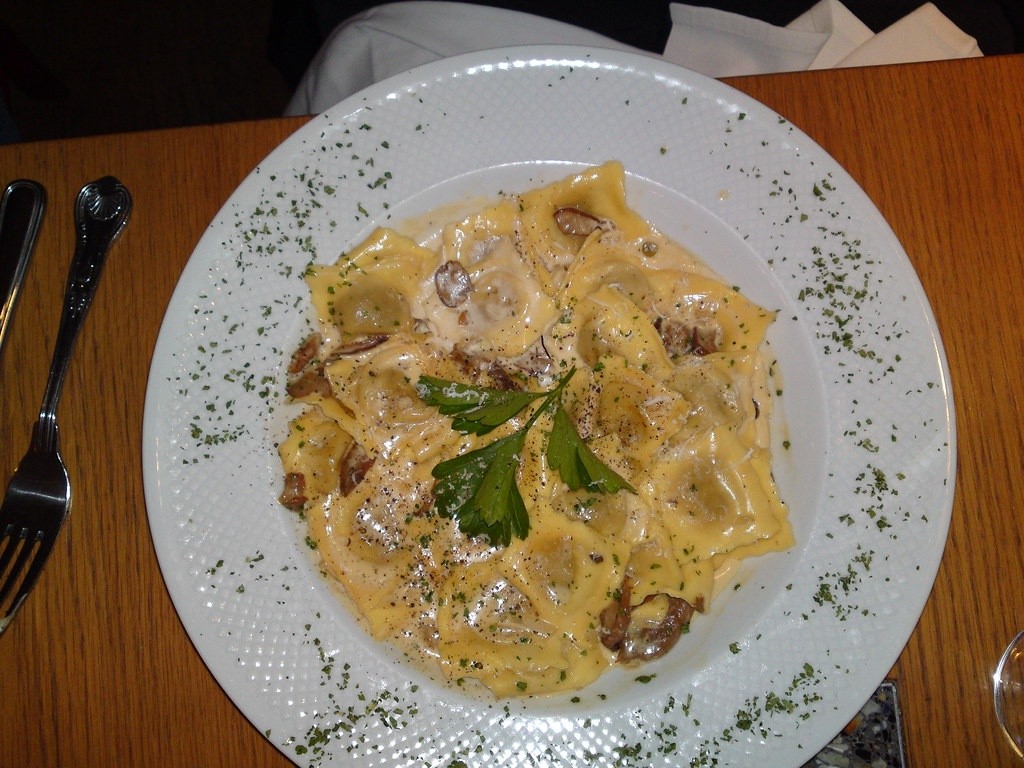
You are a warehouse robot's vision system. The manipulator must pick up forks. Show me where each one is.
[0,174,135,638]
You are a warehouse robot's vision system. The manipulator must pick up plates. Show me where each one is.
[141,46,957,768]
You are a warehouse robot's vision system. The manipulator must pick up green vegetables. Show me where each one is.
[416,364,639,550]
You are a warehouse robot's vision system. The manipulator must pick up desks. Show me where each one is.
[0,54,1023,766]
